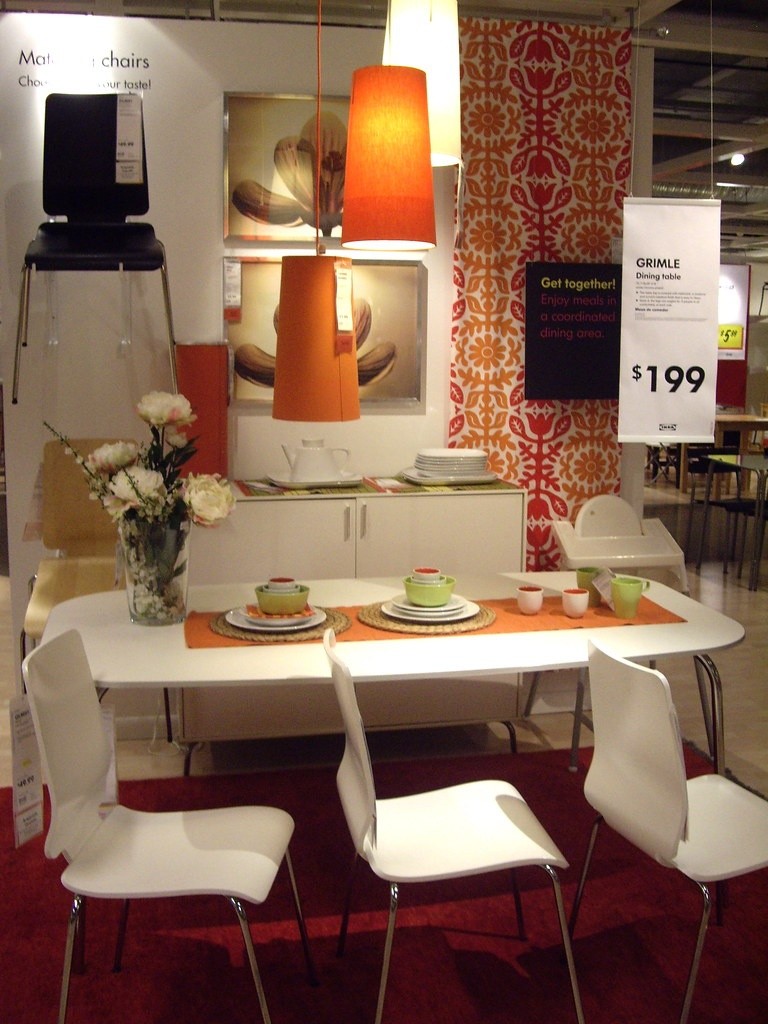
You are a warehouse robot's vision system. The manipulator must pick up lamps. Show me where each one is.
[272,1,362,423]
[341,0,437,250]
[384,0,462,167]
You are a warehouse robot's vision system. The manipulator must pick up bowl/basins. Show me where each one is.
[402,567,456,607]
[254,576,310,616]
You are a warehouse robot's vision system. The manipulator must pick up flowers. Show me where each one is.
[41,391,235,619]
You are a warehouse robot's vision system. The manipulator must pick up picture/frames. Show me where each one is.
[223,90,352,241]
[221,256,422,406]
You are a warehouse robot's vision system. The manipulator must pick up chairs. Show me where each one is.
[322,627,587,1023]
[569,634,767,1024]
[21,628,316,1024]
[524,494,717,772]
[9,93,178,405]
[18,436,172,742]
[684,447,768,591]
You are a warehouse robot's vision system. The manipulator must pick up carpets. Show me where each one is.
[0,734,767,1024]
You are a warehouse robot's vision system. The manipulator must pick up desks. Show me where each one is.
[39,570,745,777]
[679,413,768,502]
[695,455,768,592]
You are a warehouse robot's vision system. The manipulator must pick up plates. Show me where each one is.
[225,604,327,632]
[401,448,498,485]
[267,469,364,489]
[380,593,480,622]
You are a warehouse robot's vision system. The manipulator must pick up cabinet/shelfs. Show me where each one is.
[166,470,529,778]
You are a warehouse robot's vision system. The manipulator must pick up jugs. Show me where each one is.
[280,437,351,482]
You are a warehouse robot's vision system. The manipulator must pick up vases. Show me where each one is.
[115,515,190,625]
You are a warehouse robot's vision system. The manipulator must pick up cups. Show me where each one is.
[576,566,616,607]
[610,577,651,618]
[515,586,544,615]
[561,588,590,618]
